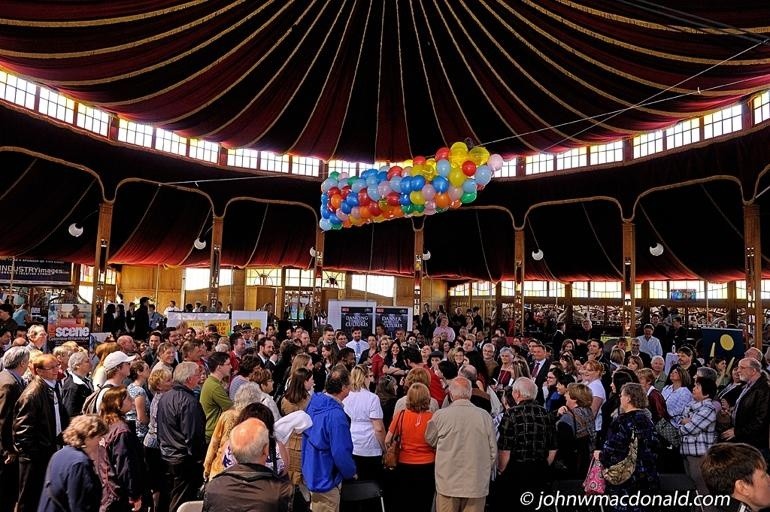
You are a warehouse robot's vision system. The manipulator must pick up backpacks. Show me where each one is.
[82,383,113,416]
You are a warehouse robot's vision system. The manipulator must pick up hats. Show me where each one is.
[243,324,253,331]
[429,352,444,357]
[103,351,137,372]
[233,325,242,331]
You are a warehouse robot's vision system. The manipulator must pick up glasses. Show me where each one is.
[545,376,556,379]
[224,361,232,365]
[43,363,61,371]
[618,393,627,398]
[37,333,48,337]
[195,372,203,377]
[736,365,753,369]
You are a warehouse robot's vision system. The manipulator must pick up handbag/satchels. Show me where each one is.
[582,456,608,495]
[381,410,406,470]
[655,414,685,449]
[554,410,583,484]
[143,425,159,449]
[602,425,638,486]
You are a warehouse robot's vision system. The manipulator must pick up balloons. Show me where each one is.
[319,141,504,231]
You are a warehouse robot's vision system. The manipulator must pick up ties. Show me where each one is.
[733,381,752,412]
[718,384,735,400]
[532,363,540,376]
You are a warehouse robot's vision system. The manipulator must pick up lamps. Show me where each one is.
[194,226,212,250]
[309,246,317,256]
[422,248,432,260]
[525,223,544,261]
[638,213,664,257]
[68,210,98,237]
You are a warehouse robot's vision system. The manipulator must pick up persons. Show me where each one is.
[103,296,231,369]
[124,360,151,512]
[384,384,436,467]
[63,352,89,416]
[343,364,386,481]
[301,366,357,512]
[202,418,310,512]
[91,340,117,393]
[497,377,558,480]
[36,413,109,512]
[156,360,206,512]
[11,354,69,512]
[282,369,315,500]
[0,294,76,382]
[198,351,232,442]
[95,351,134,414]
[203,382,260,480]
[0,347,30,464]
[144,366,173,461]
[223,401,284,475]
[425,375,498,511]
[98,386,142,512]
